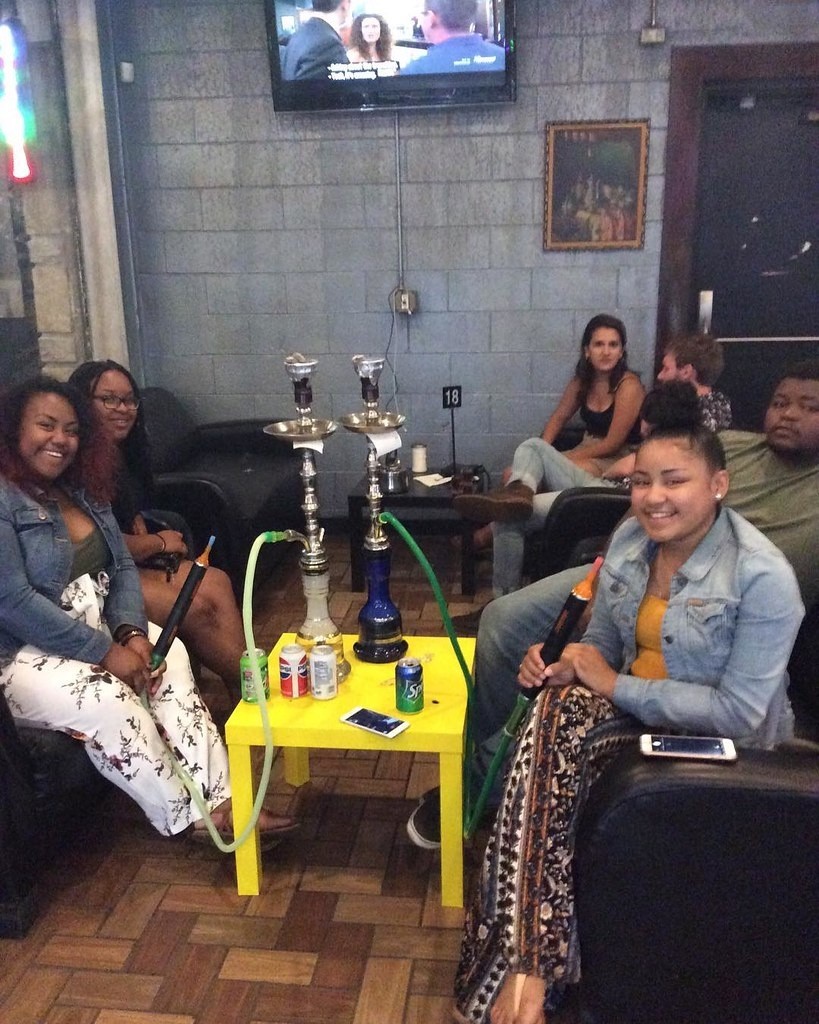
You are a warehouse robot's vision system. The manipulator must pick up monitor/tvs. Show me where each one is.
[263,0,517,115]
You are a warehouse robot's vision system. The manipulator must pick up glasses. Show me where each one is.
[93,395,140,411]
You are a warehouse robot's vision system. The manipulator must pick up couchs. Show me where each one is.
[0,505,203,939]
[531,427,633,567]
[542,537,819,1024]
[122,387,314,614]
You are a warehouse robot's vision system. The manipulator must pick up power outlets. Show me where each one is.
[394,290,418,314]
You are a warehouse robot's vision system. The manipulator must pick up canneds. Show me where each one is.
[394,658,425,714]
[309,645,338,700]
[279,644,308,698]
[239,649,270,703]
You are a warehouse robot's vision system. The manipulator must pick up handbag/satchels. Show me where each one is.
[120,510,183,583]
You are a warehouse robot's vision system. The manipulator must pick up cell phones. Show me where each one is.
[639,734,737,761]
[339,707,410,739]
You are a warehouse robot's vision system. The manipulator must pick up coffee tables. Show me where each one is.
[347,467,484,596]
[224,632,478,908]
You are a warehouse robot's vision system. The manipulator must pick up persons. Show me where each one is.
[0,372,303,854]
[475,312,649,550]
[453,375,805,1024]
[407,356,819,850]
[68,359,248,709]
[283,0,504,80]
[452,316,731,635]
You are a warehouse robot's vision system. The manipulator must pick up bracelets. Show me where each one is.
[154,533,167,553]
[119,628,147,646]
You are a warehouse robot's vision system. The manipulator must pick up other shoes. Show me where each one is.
[450,533,493,551]
[408,793,443,850]
[194,801,302,838]
[420,786,445,803]
[448,596,495,637]
[452,480,534,521]
[174,823,282,853]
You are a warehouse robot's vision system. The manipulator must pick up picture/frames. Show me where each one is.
[543,118,651,252]
